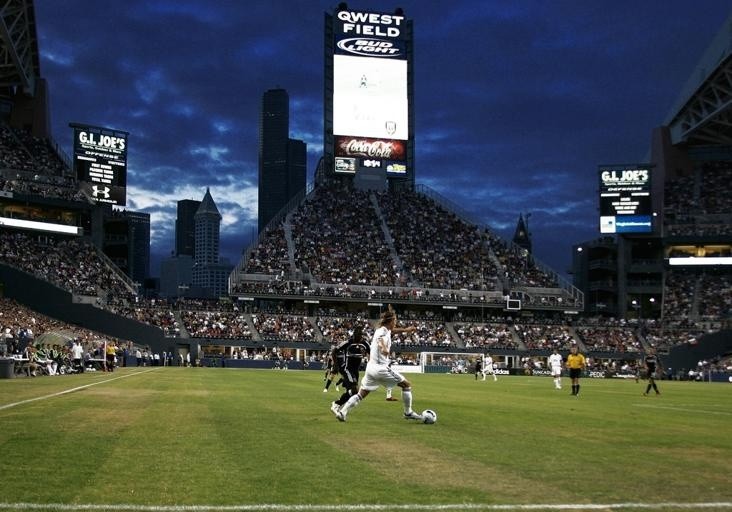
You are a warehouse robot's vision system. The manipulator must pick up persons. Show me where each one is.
[586,156,732,381]
[481,351,497,380]
[0,124,231,379]
[565,344,587,397]
[547,349,563,391]
[385,386,398,402]
[474,351,483,380]
[231,179,584,376]
[336,309,421,418]
[330,326,371,415]
[323,340,345,392]
[642,347,663,396]
[324,348,331,382]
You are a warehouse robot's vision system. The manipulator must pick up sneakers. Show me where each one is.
[402,410,422,420]
[323,377,340,392]
[386,396,397,401]
[330,401,346,421]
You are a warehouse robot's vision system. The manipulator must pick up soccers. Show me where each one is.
[421,409,437,424]
